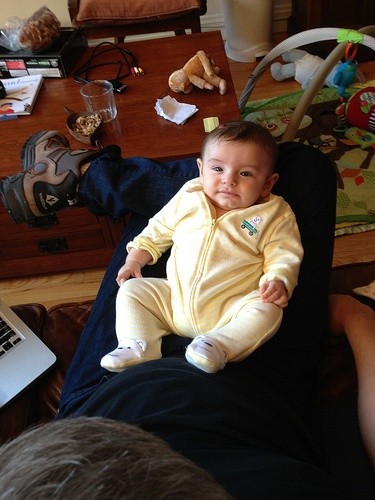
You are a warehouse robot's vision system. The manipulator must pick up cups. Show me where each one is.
[79,80,117,122]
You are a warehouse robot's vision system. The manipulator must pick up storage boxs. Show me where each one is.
[0,26,87,80]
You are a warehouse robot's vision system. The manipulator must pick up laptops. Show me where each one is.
[0,301,57,408]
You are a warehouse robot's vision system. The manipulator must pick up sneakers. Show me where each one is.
[0,130,119,223]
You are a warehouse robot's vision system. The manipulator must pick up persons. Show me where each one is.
[0,131,375,500]
[100,121,304,374]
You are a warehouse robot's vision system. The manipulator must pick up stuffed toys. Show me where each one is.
[168,50,226,95]
[270,49,344,91]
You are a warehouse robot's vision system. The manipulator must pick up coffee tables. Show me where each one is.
[0,29,242,279]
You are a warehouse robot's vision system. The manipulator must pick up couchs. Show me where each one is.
[0,260,375,446]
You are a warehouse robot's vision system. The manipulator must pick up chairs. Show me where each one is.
[66,0,208,45]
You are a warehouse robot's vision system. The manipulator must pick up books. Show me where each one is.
[0,74,44,116]
